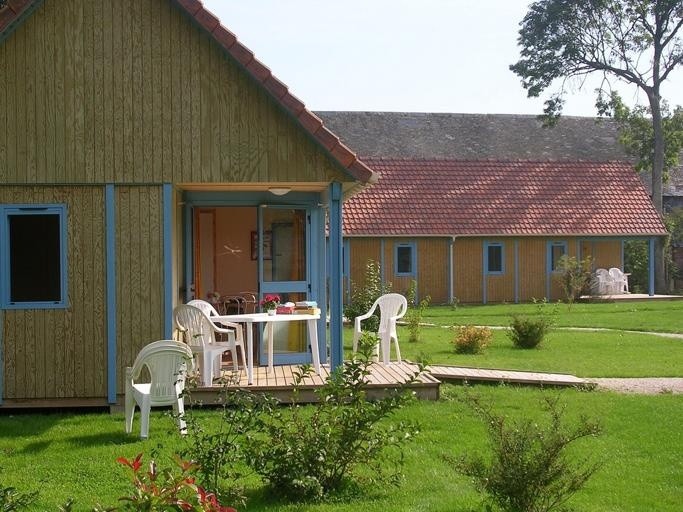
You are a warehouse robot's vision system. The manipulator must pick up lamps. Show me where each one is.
[267,188,291,196]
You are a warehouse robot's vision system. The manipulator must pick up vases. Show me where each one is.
[267,310,276,316]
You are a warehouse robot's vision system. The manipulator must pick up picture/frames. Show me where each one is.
[251,230,273,261]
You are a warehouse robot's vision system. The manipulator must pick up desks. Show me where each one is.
[208,313,321,385]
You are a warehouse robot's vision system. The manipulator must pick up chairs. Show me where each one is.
[353,293,407,365]
[595,267,632,295]
[124,290,259,440]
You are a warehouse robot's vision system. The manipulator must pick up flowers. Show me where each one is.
[258,293,281,312]
